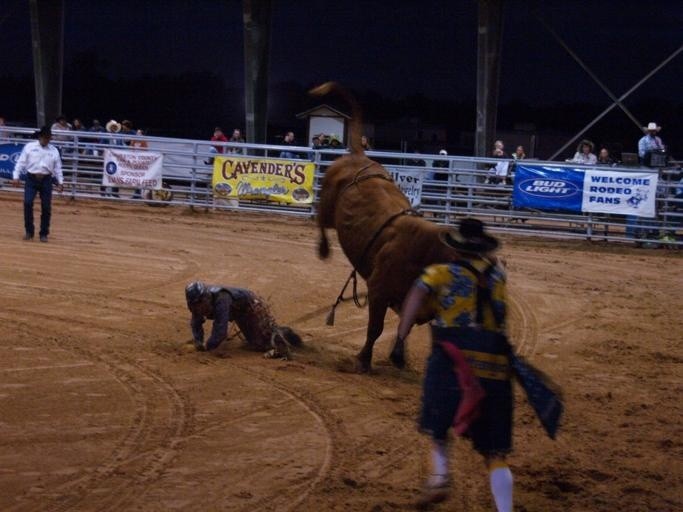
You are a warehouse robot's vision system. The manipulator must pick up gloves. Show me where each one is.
[390,338,405,370]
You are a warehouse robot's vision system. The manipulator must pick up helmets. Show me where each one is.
[185,281,207,305]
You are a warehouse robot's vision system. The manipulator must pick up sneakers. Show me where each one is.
[23,232,34,240]
[274,334,288,357]
[40,235,47,241]
[285,328,301,346]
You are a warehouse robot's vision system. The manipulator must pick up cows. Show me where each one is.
[309,80,509,373]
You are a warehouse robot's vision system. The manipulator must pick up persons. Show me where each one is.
[512,145,527,160]
[565,140,598,166]
[485,140,510,184]
[597,146,620,168]
[228,129,248,155]
[638,121,666,167]
[280,132,300,159]
[307,134,379,162]
[390,218,564,512]
[51,114,148,200]
[210,128,227,152]
[432,149,450,180]
[13,124,64,242]
[181,279,303,360]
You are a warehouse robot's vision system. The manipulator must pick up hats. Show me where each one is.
[577,140,594,152]
[439,149,448,155]
[34,126,56,136]
[106,120,121,133]
[439,219,500,251]
[642,122,661,133]
